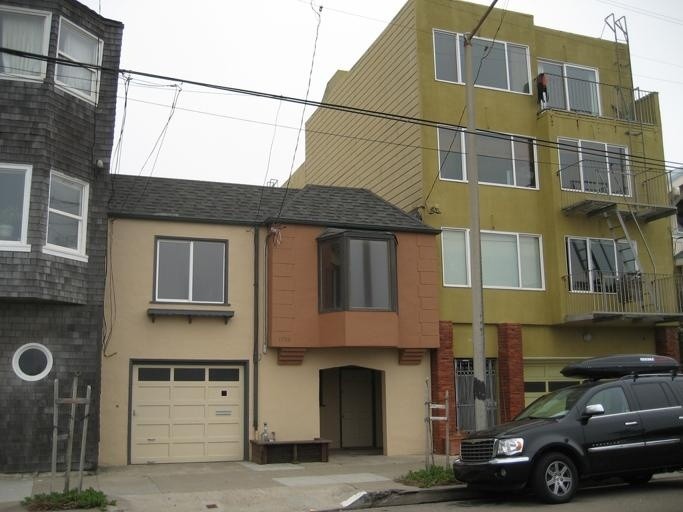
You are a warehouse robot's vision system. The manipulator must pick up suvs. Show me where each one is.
[451,352,683,504]
